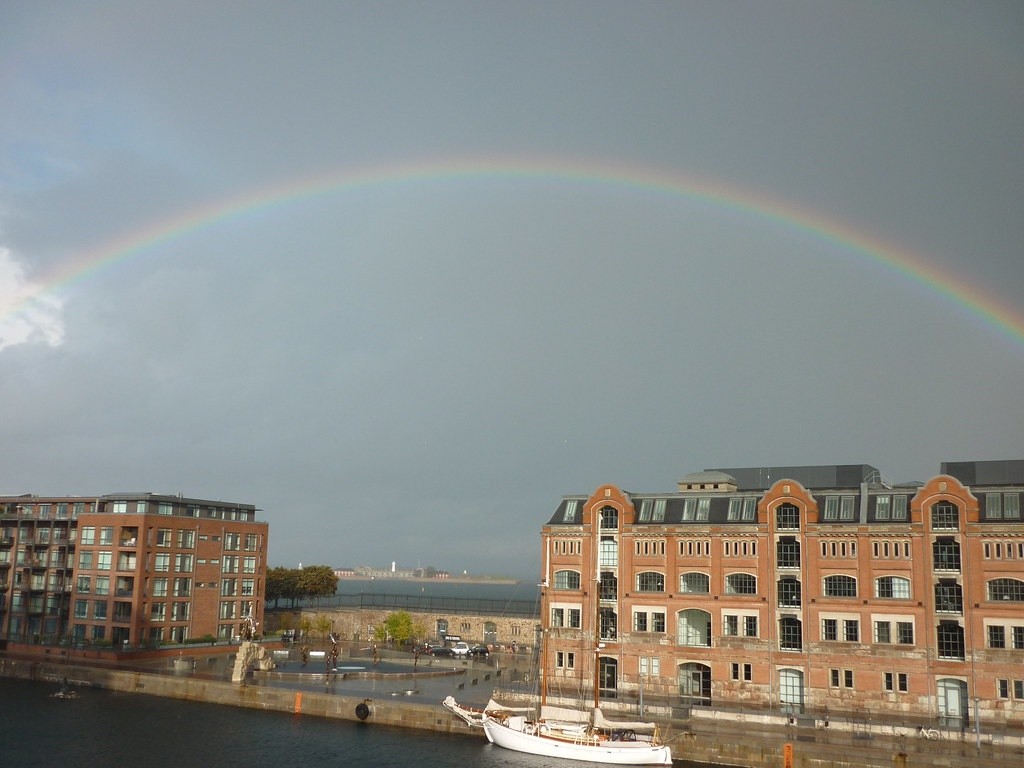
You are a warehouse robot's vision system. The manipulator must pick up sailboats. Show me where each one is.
[442,510,673,767]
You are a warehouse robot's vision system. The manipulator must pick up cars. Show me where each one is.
[427,645,454,657]
[467,645,490,657]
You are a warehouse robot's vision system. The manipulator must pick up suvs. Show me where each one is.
[449,642,469,656]
